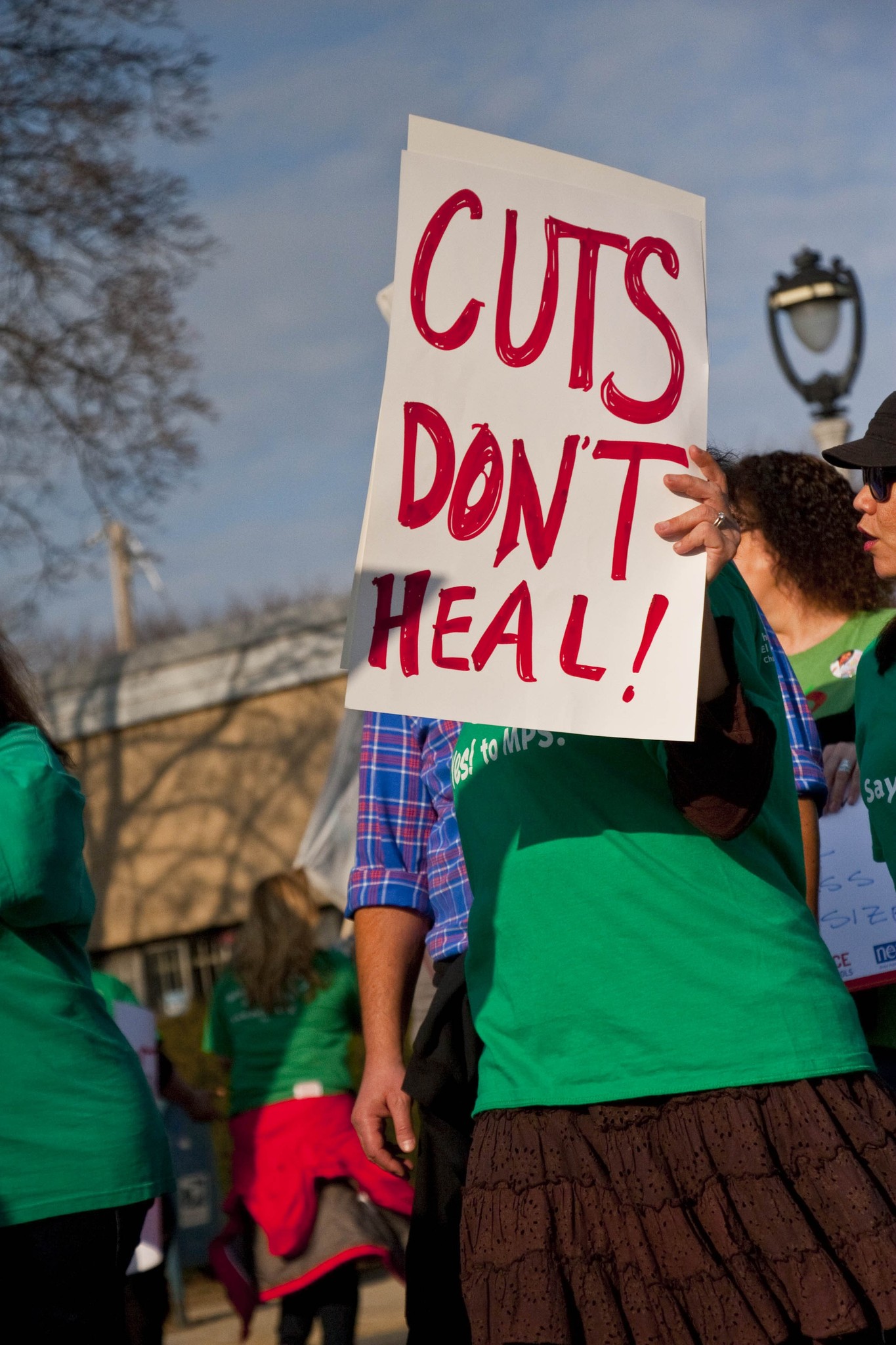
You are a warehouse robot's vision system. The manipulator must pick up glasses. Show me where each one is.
[863,467,896,502]
[732,515,767,531]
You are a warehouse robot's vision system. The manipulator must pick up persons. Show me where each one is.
[0,652,174,1345]
[91,869,410,1345]
[706,389,896,1105]
[346,446,896,1345]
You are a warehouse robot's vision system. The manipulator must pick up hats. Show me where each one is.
[822,391,896,469]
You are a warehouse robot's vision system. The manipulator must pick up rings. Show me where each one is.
[839,758,853,774]
[713,510,726,529]
[713,512,730,529]
[837,759,853,774]
